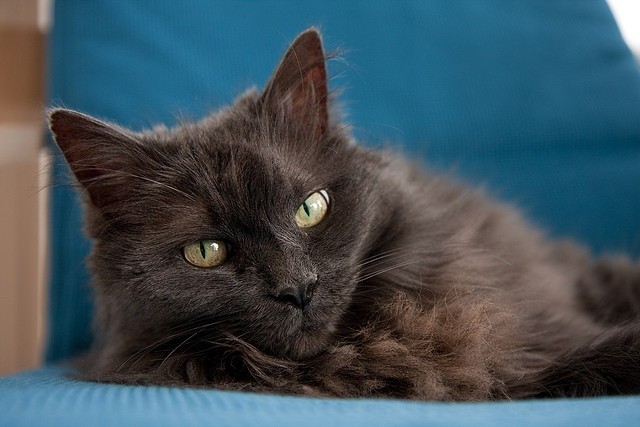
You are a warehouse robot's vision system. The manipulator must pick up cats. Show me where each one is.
[48,27,640,403]
